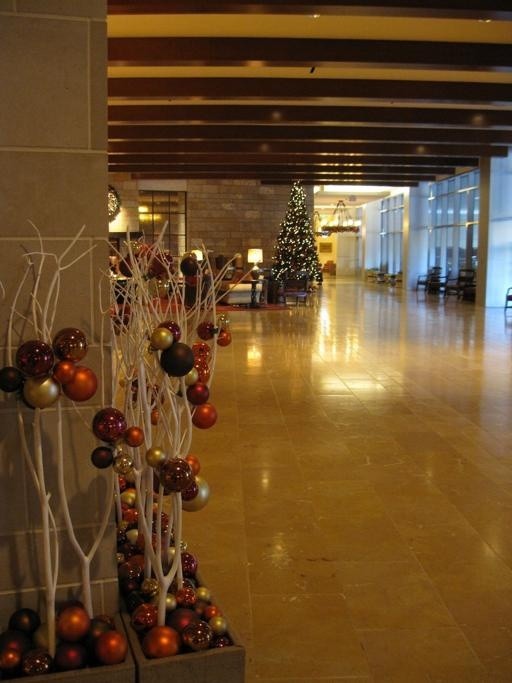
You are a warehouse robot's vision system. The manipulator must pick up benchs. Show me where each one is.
[219,280,263,305]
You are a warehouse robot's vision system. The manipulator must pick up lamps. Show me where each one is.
[322,200,358,232]
[248,249,263,279]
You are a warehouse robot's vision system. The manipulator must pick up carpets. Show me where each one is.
[115,297,292,313]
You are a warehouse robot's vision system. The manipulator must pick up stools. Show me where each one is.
[284,292,307,306]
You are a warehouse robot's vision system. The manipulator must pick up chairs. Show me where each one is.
[444,269,475,299]
[416,266,451,293]
